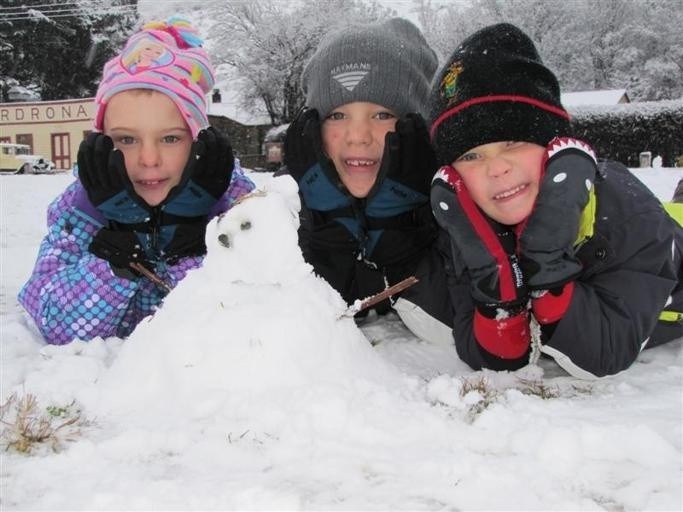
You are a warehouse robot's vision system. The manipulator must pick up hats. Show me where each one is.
[299,17,439,131]
[93,17,217,142]
[427,22,574,166]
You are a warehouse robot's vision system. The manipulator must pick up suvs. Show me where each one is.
[1,142,51,175]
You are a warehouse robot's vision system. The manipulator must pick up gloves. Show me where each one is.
[77,125,234,281]
[282,105,439,273]
[516,137,598,291]
[430,165,530,307]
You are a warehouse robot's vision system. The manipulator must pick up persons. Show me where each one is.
[280,16,456,354]
[426,19,683,380]
[18,15,256,344]
